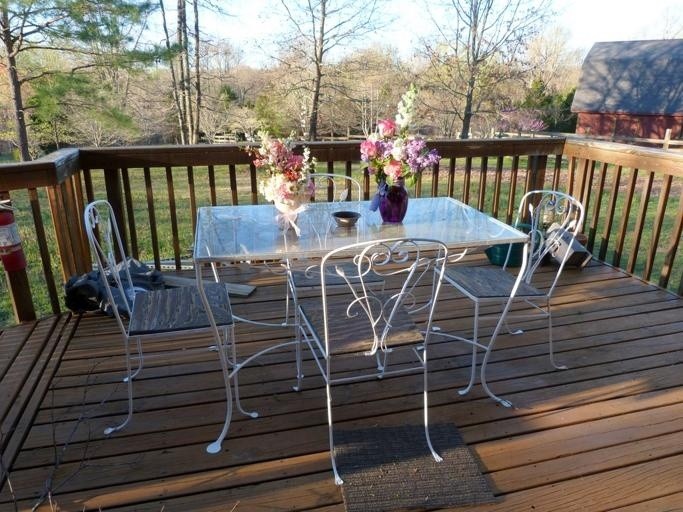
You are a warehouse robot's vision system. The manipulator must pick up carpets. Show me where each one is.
[332,422,496,511]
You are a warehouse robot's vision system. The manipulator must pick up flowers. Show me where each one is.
[355,78,441,187]
[239,124,322,208]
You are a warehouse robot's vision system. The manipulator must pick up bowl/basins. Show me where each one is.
[332,212,361,227]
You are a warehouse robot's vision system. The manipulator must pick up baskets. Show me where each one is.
[485,222,547,266]
